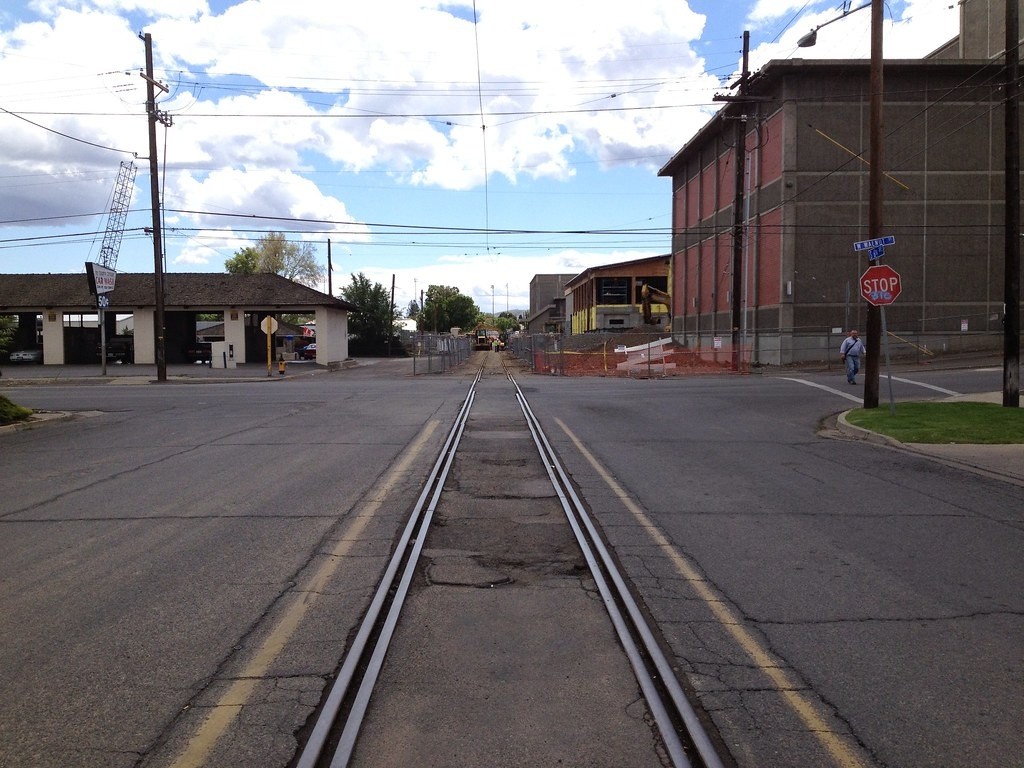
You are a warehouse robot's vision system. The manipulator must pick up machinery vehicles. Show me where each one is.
[641,284,672,331]
[474,329,490,351]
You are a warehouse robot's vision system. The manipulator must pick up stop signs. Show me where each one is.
[859,264,902,306]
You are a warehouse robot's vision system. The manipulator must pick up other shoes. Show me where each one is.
[851,381,856,385]
[847,380,851,382]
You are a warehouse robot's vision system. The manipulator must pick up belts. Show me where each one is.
[848,354,859,357]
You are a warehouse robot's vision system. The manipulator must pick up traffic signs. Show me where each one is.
[866,244,884,261]
[853,235,896,252]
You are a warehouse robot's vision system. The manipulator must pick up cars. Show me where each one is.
[305,344,315,360]
[188,343,212,364]
[10,350,43,364]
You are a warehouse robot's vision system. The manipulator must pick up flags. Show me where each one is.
[304,327,314,336]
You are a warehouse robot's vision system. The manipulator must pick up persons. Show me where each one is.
[840,330,866,385]
[490,339,500,352]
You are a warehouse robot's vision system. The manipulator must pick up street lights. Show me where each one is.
[797,3,884,408]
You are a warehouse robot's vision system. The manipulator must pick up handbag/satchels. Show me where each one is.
[843,355,847,364]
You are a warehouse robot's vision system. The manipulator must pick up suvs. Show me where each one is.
[96,335,133,364]
